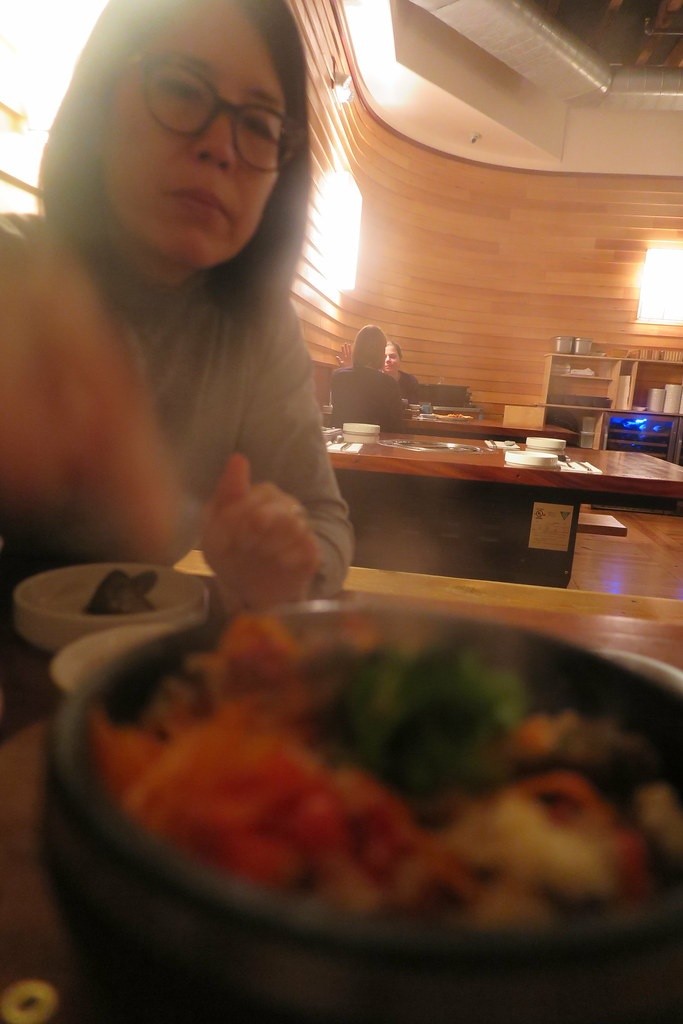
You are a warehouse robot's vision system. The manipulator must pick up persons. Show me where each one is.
[0,0,359,617]
[330,325,422,433]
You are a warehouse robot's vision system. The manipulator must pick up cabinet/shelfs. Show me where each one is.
[590,412,683,516]
[534,353,683,410]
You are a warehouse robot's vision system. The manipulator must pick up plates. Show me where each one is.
[11,562,210,655]
[633,383,683,414]
[443,415,473,421]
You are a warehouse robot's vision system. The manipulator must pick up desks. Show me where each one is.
[322,412,579,446]
[433,407,485,420]
[0,549,683,1024]
[327,431,683,589]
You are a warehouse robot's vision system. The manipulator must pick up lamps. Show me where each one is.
[330,55,356,104]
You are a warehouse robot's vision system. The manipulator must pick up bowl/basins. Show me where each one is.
[504,450,558,468]
[526,436,566,451]
[49,623,183,694]
[343,422,380,436]
[39,600,683,1024]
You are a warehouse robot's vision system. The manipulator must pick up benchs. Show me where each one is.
[578,513,629,537]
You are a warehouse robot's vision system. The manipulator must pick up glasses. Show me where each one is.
[114,44,306,171]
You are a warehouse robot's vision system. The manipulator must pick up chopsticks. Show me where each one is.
[576,460,591,470]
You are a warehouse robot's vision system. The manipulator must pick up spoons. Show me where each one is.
[558,454,575,469]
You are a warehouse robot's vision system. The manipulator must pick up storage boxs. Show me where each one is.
[576,415,596,431]
[548,392,564,404]
[577,432,595,448]
[564,394,576,406]
[430,383,471,406]
[576,395,584,405]
[551,336,574,354]
[417,385,432,401]
[594,397,611,409]
[572,337,595,355]
[583,396,593,407]
